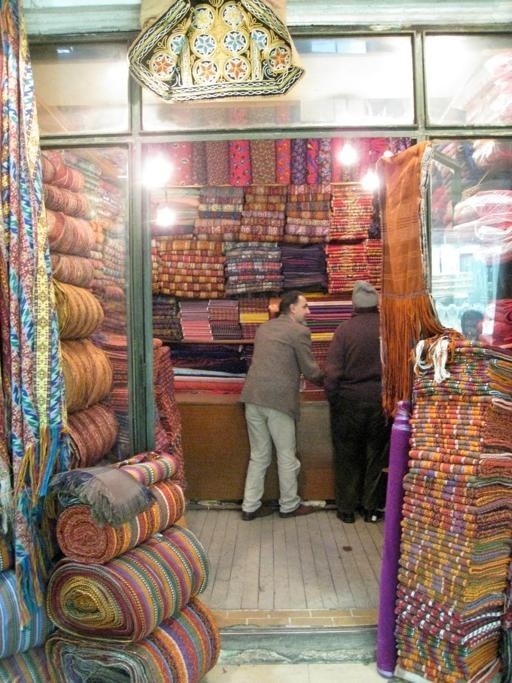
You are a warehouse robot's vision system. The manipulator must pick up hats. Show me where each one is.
[351,279,379,309]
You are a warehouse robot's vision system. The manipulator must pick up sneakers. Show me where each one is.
[336,510,355,524]
[364,511,384,523]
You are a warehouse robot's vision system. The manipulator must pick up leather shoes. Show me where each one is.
[279,504,315,519]
[241,505,275,522]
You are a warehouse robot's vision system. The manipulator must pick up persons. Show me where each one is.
[459,310,487,343]
[237,289,323,519]
[323,281,385,522]
